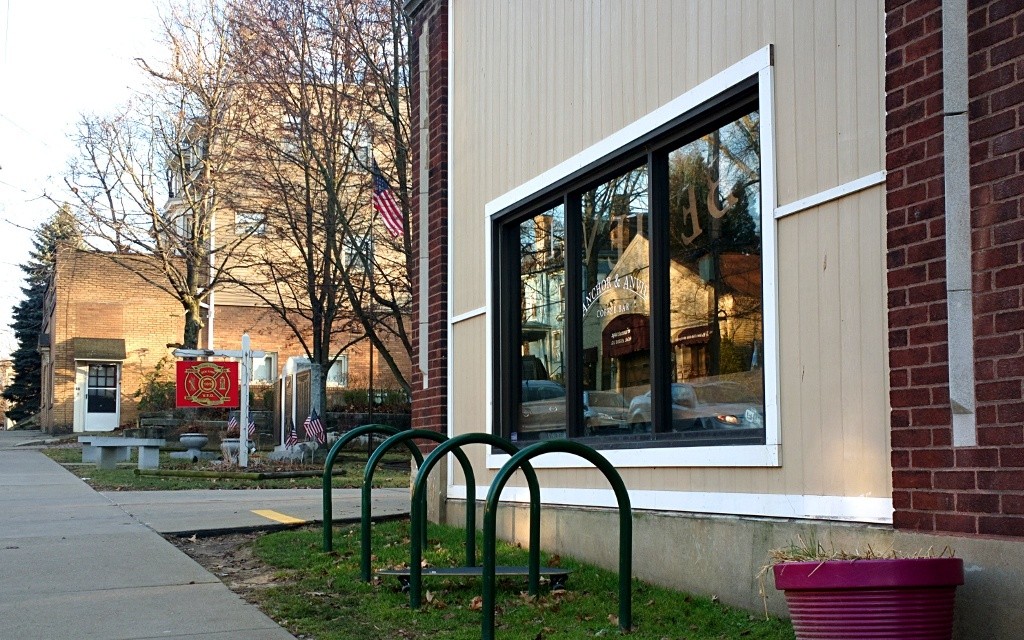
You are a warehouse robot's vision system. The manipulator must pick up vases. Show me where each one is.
[773,559,964,640]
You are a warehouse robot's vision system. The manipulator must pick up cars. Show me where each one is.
[625,382,765,428]
[583,391,629,437]
[519,381,567,430]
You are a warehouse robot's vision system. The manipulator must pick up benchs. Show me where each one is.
[78,436,166,469]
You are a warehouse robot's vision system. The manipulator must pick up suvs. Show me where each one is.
[522,355,548,380]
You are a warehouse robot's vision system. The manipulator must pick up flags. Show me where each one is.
[372,159,404,238]
[303,408,326,445]
[285,421,298,450]
[248,412,255,436]
[228,414,238,433]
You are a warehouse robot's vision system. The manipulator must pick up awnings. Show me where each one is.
[73,337,126,362]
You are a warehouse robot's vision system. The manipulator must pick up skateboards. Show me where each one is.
[376,567,574,593]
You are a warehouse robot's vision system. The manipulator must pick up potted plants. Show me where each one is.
[180,420,253,459]
